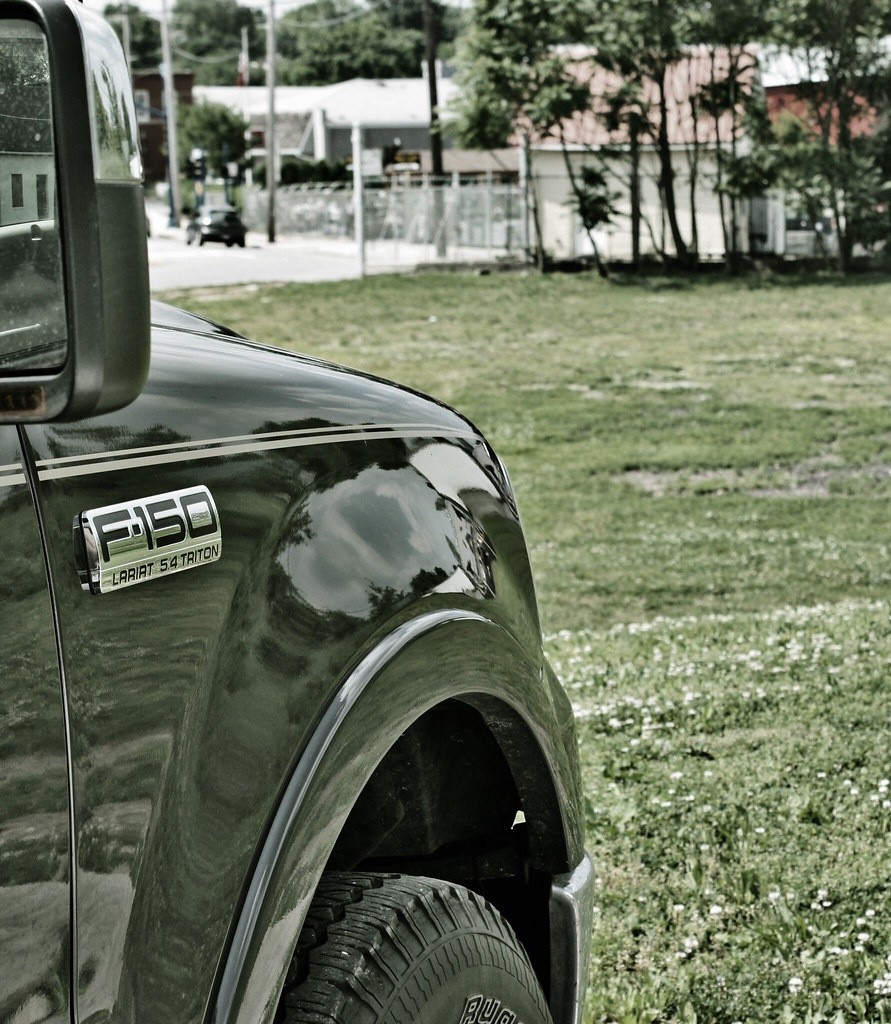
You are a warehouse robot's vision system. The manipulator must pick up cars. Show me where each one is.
[185,205,249,250]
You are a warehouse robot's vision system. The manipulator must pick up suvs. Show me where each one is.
[0,1,597,1024]
